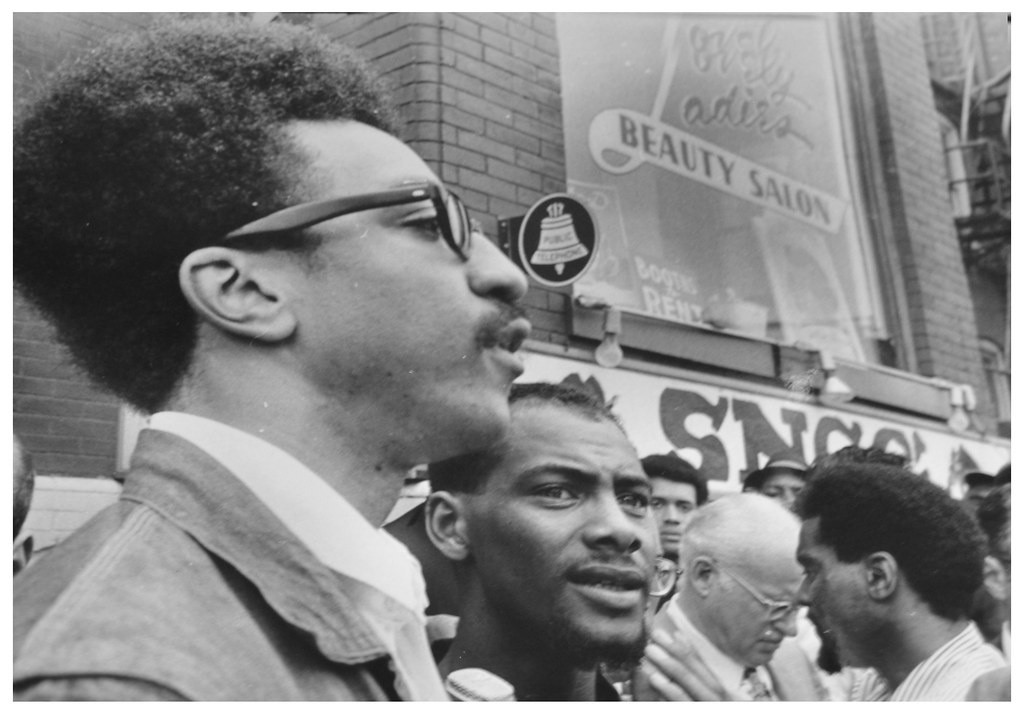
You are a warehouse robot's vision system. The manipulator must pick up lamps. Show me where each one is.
[796,339,854,405]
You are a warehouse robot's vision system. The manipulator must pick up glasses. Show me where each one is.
[709,559,793,623]
[227,178,473,264]
[650,557,682,598]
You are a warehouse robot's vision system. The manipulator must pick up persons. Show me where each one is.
[629,491,828,702]
[422,382,661,702]
[809,446,906,471]
[12,432,35,576]
[13,19,529,703]
[641,461,1010,701]
[600,504,685,701]
[961,461,1011,658]
[641,455,711,563]
[740,452,812,512]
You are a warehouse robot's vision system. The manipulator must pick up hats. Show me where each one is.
[963,472,995,488]
[743,451,813,487]
